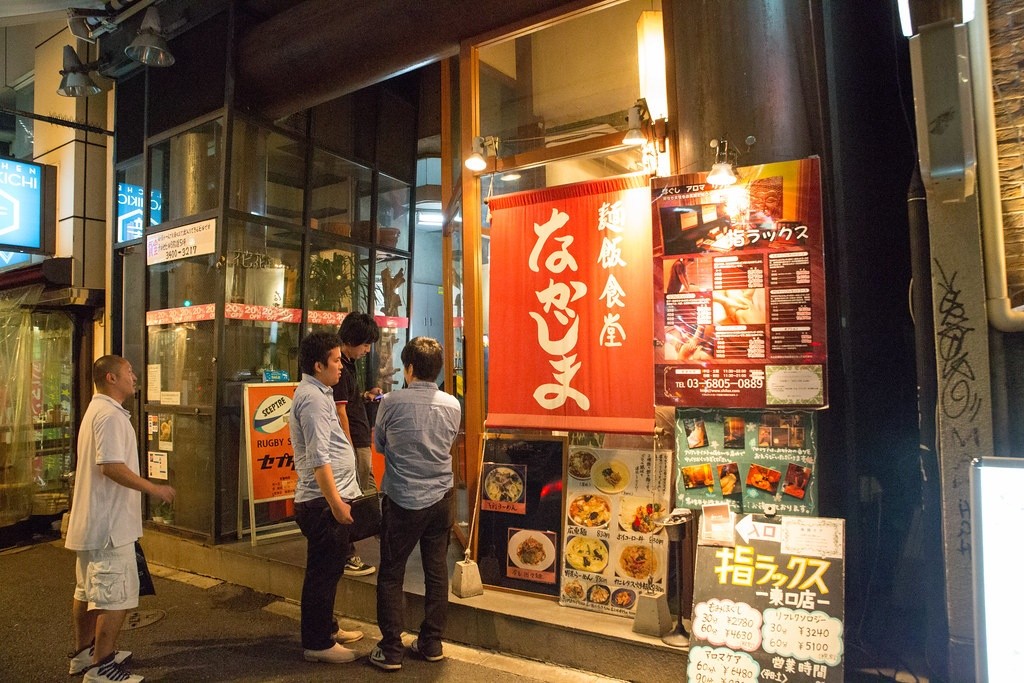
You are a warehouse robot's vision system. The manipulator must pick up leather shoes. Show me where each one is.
[329,628,364,646]
[304,643,359,664]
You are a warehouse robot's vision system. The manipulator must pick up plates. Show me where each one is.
[562,578,588,602]
[585,581,610,605]
[610,586,636,609]
[617,493,670,536]
[507,530,557,571]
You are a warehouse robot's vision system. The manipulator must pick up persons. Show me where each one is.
[367,337,461,667]
[291,331,365,664]
[667,257,694,293]
[665,326,713,360]
[331,312,382,576]
[64,355,175,682]
[714,289,765,324]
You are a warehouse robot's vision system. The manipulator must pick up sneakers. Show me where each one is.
[68,638,133,674]
[411,638,444,662]
[343,556,377,577]
[370,646,402,669]
[82,652,145,683]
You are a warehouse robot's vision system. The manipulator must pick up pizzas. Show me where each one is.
[566,536,609,572]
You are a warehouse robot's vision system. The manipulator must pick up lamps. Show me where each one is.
[620,107,648,146]
[635,10,670,121]
[56,43,112,98]
[706,140,739,186]
[464,135,489,173]
[124,4,174,68]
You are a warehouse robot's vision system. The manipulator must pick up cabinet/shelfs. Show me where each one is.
[142,104,418,543]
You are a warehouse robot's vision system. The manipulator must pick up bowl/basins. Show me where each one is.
[564,535,610,575]
[567,489,613,528]
[590,457,631,495]
[612,539,666,583]
[482,464,525,504]
[566,446,600,481]
